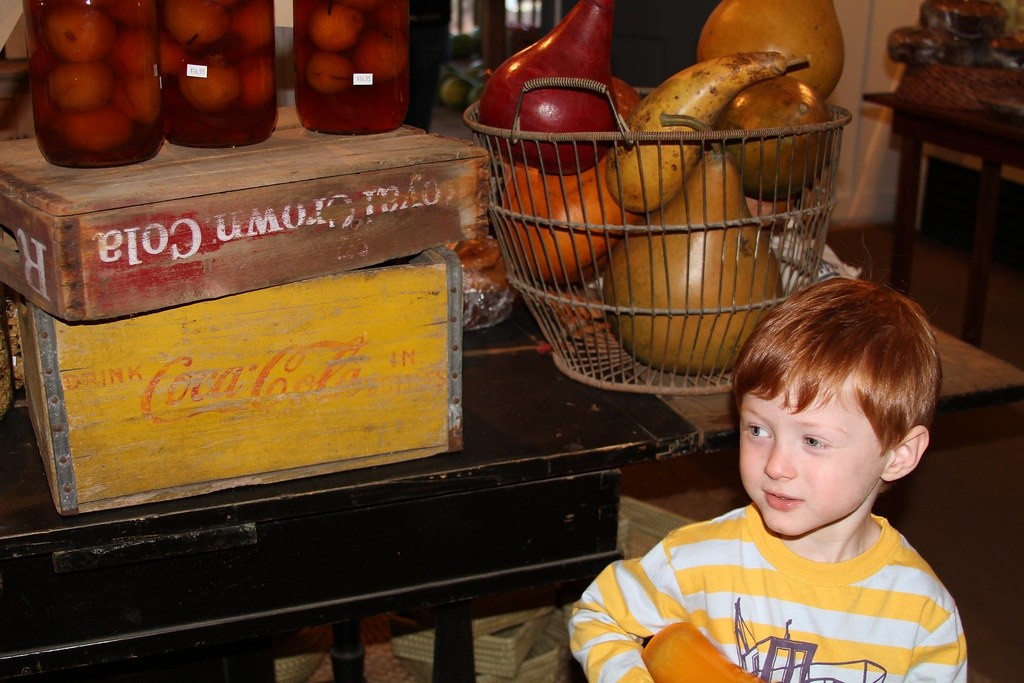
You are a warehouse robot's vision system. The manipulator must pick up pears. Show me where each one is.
[42,0,407,153]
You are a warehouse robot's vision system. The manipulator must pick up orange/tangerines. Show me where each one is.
[441,30,489,106]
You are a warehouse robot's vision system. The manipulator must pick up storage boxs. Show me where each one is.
[12,247,465,516]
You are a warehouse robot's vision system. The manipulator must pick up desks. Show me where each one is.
[864,90,1024,346]
[1,290,1023,682]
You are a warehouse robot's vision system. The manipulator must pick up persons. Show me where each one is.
[568,277,968,683]
